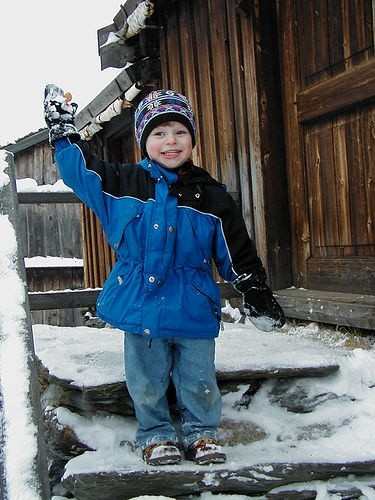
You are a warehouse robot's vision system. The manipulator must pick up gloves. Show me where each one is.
[230,267,285,332]
[42,84,83,143]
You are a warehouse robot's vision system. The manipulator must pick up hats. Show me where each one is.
[133,88,197,156]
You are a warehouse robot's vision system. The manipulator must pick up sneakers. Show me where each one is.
[184,437,228,463]
[142,441,180,464]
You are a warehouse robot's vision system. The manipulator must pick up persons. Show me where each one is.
[42,83,287,466]
